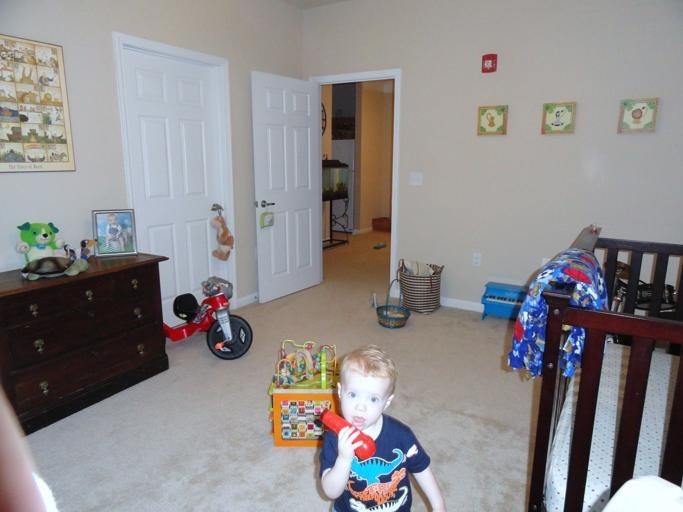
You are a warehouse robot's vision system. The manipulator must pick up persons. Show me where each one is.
[316,341,449,512]
[99,213,128,253]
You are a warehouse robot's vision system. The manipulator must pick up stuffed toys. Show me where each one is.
[78,237,102,263]
[20,255,91,283]
[12,219,67,264]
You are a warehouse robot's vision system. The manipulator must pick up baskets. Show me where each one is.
[376,279,409,328]
[396,259,444,315]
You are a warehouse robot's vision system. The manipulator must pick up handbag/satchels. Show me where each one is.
[609,280,675,345]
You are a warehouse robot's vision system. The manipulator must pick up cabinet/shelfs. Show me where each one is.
[1,263,169,438]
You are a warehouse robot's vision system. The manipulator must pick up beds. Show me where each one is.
[528,227,683,512]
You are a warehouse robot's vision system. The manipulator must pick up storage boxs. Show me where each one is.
[268,374,341,447]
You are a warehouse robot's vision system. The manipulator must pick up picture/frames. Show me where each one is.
[541,100,576,135]
[0,34,77,173]
[617,97,659,134]
[477,104,508,136]
[91,209,139,259]
